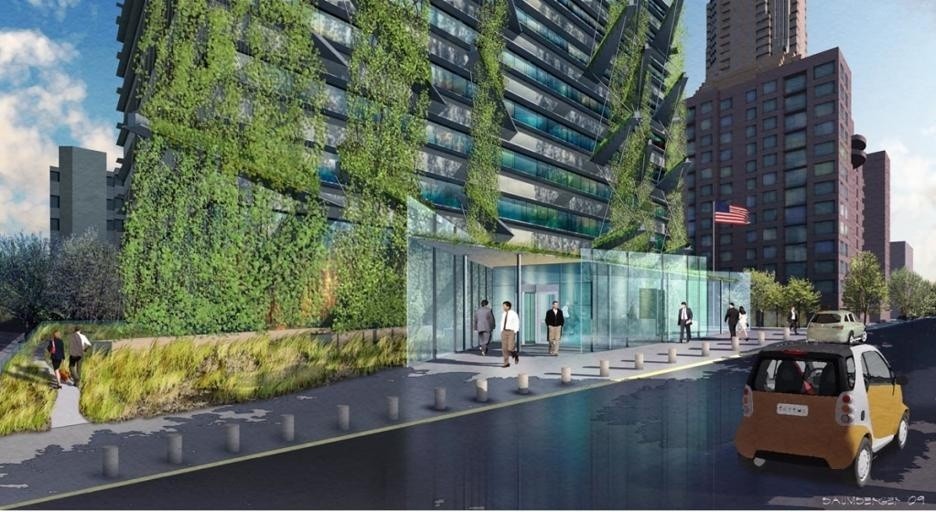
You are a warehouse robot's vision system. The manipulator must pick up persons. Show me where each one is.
[737,306,750,340]
[545,300,565,356]
[498,301,520,369]
[723,302,739,336]
[68,326,92,386]
[46,329,66,389]
[472,299,496,356]
[787,305,800,335]
[677,301,693,343]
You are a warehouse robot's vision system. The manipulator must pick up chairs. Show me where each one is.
[774,359,852,396]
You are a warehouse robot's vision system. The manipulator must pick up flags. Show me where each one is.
[714,200,752,226]
